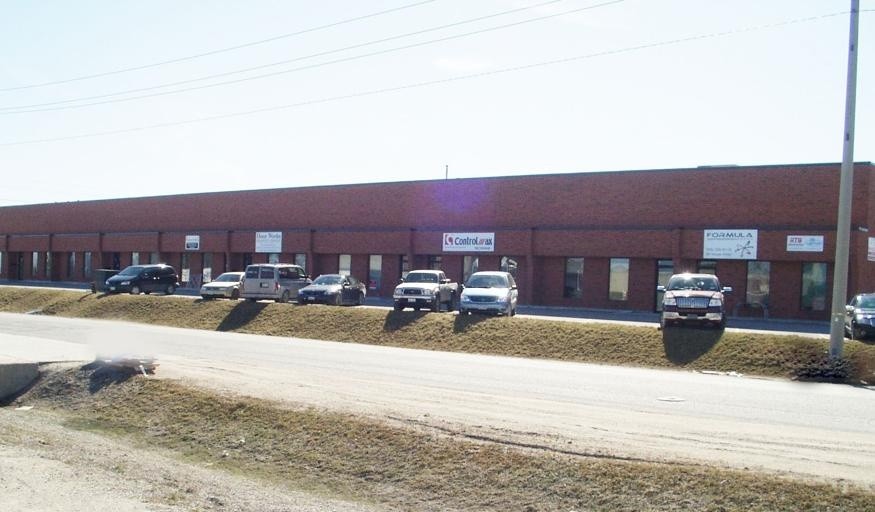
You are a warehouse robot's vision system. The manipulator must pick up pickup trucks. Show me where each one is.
[392,270,458,312]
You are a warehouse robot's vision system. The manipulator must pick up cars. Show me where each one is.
[199,263,366,306]
[459,271,519,317]
[845,293,875,341]
[656,273,733,328]
[103,264,180,294]
